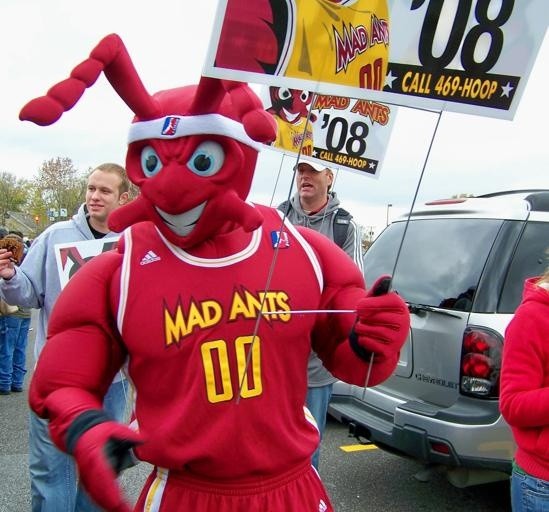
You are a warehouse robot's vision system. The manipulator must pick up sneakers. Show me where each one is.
[0,386,23,396]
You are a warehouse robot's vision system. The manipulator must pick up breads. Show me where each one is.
[0,234,24,263]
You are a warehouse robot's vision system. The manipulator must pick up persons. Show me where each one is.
[0,226,32,266]
[268,157,364,473]
[0,238,31,395]
[498,254,549,512]
[0,162,134,512]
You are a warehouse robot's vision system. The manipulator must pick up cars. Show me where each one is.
[327,189,549,488]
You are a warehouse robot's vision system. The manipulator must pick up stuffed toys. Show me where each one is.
[19,30,411,511]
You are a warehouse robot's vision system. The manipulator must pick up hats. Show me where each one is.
[292,159,334,175]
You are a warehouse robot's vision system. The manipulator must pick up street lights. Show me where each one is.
[387,203,392,224]
